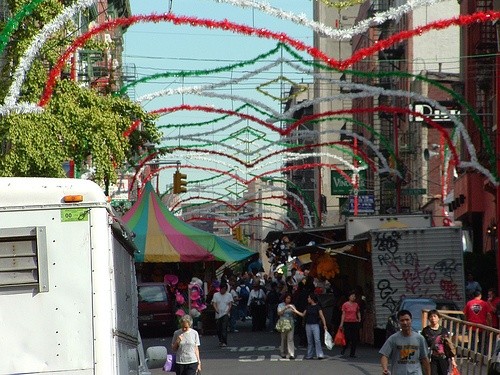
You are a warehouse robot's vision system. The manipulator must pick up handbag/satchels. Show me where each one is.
[443,328,456,358]
[325,329,335,350]
[275,318,293,333]
[334,327,346,346]
[248,301,258,314]
[452,367,462,375]
[163,352,177,372]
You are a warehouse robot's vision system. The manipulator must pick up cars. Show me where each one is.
[136,282,180,335]
[384,297,464,351]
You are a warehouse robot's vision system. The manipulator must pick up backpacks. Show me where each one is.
[239,286,249,299]
[267,289,278,304]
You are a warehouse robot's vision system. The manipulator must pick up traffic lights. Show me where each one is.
[172,173,189,194]
[233,225,244,241]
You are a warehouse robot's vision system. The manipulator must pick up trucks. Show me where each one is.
[0,177,149,374]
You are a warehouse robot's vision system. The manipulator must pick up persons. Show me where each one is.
[212,283,233,346]
[277,293,296,359]
[421,310,457,375]
[211,235,315,332]
[331,275,361,358]
[172,315,201,375]
[380,310,431,375]
[465,272,500,358]
[292,294,327,359]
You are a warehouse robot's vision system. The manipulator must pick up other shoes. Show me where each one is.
[219,340,227,347]
[349,354,358,358]
[304,355,314,360]
[282,355,286,359]
[317,356,328,360]
[289,356,295,360]
[340,348,346,354]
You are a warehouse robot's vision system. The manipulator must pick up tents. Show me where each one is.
[123,180,259,277]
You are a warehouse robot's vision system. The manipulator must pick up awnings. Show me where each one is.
[264,225,345,243]
[291,238,369,261]
